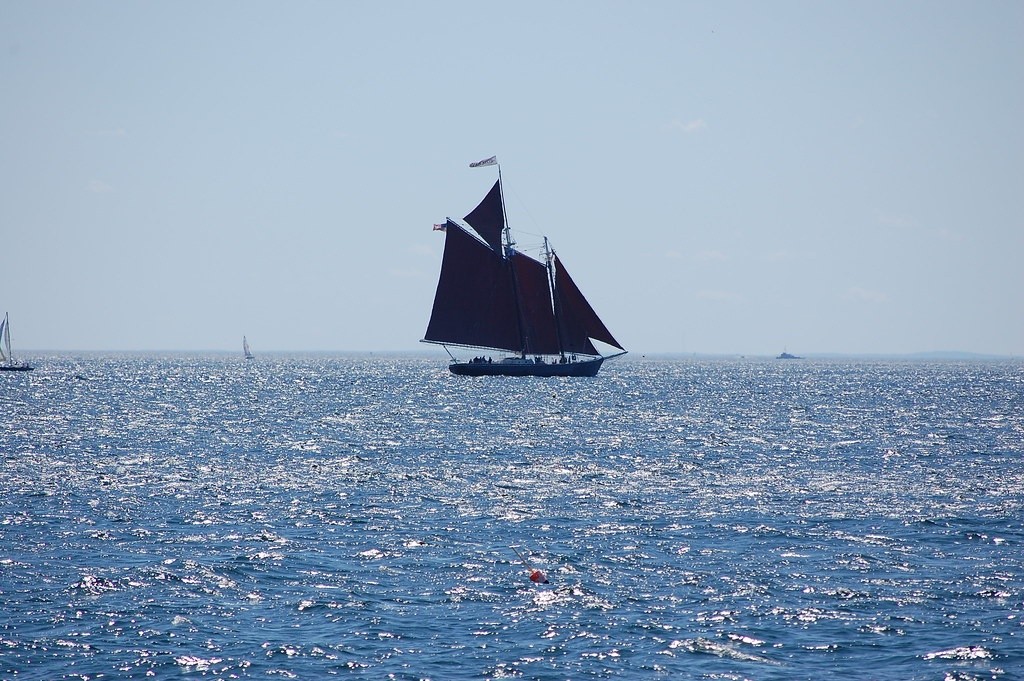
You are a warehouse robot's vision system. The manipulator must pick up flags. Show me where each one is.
[469,156,498,167]
[433,223,447,232]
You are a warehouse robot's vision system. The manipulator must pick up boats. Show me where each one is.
[776,353,801,359]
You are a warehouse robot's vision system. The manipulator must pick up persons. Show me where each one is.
[469,356,492,364]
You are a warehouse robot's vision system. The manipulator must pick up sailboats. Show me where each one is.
[420,155,628,377]
[242,336,255,359]
[0,312,35,371]
